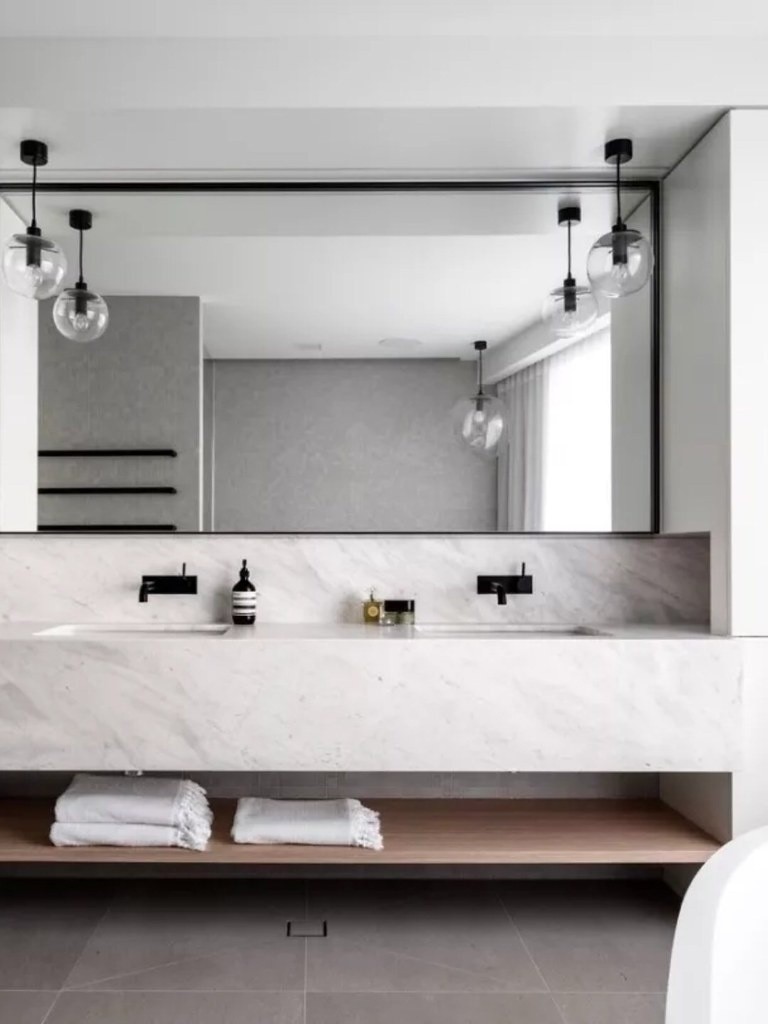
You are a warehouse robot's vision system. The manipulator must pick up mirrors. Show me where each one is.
[0,180,660,538]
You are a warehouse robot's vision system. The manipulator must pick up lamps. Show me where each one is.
[587,139,655,297]
[52,209,109,343]
[2,140,69,299]
[450,339,508,451]
[540,208,598,335]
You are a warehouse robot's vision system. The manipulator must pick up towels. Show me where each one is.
[231,794,385,851]
[46,773,214,850]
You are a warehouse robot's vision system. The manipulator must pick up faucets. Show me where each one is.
[486,579,509,606]
[136,581,159,603]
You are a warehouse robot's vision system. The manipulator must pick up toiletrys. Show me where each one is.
[230,558,258,625]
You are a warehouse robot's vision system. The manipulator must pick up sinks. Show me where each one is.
[412,622,611,639]
[34,622,232,640]
